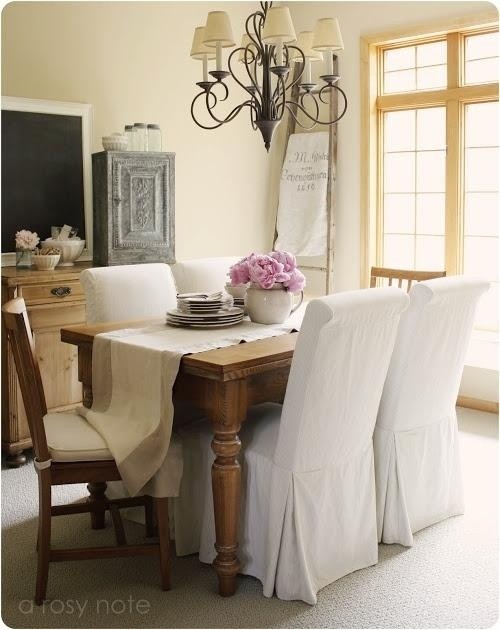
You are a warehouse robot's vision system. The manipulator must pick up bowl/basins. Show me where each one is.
[102,136,129,149]
[31,255,61,270]
[224,281,248,297]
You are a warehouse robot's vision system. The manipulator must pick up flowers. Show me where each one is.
[14,230,39,250]
[229,253,307,291]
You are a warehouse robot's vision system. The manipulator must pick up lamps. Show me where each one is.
[190,2,348,155]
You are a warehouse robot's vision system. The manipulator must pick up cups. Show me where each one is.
[247,282,303,325]
[16,247,32,268]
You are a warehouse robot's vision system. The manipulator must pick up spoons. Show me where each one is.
[177,290,224,300]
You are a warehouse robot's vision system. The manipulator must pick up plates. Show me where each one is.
[164,292,244,329]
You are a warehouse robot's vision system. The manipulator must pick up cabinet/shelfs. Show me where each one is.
[93,152,177,265]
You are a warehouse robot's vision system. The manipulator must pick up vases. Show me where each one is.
[102,133,128,152]
[16,251,31,268]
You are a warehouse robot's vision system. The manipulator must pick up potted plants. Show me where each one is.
[32,246,62,271]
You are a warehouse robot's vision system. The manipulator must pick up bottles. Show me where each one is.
[124,123,161,151]
